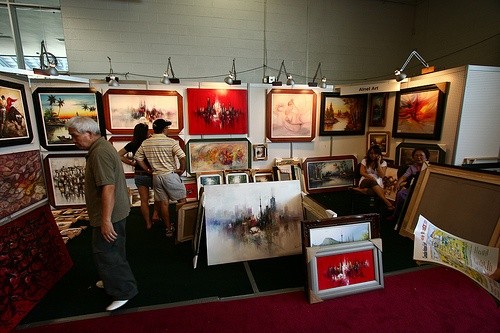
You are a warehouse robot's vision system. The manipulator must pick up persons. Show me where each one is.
[64,116,139,311]
[133,119,186,237]
[358,144,397,212]
[118,123,164,229]
[393,145,431,207]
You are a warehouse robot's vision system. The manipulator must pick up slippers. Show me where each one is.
[152,217,162,222]
[146,222,154,232]
[387,205,395,210]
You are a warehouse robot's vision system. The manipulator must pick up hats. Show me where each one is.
[153,119,171,129]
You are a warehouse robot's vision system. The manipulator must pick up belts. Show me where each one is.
[134,171,149,176]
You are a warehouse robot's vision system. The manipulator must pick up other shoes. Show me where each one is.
[106,300,127,310]
[96,281,105,288]
[165,223,175,237]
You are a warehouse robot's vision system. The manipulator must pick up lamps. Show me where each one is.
[225,59,239,85]
[40,40,59,75]
[395,50,430,82]
[313,62,326,88]
[108,57,118,86]
[275,60,295,85]
[160,57,180,84]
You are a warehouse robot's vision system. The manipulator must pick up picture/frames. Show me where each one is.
[0,79,500,304]
